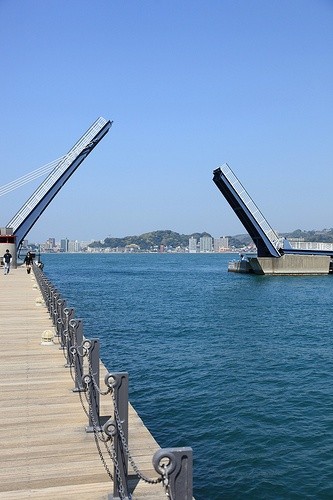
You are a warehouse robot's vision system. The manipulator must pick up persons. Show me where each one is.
[24,252,33,275]
[2,249,13,275]
[240,254,245,261]
